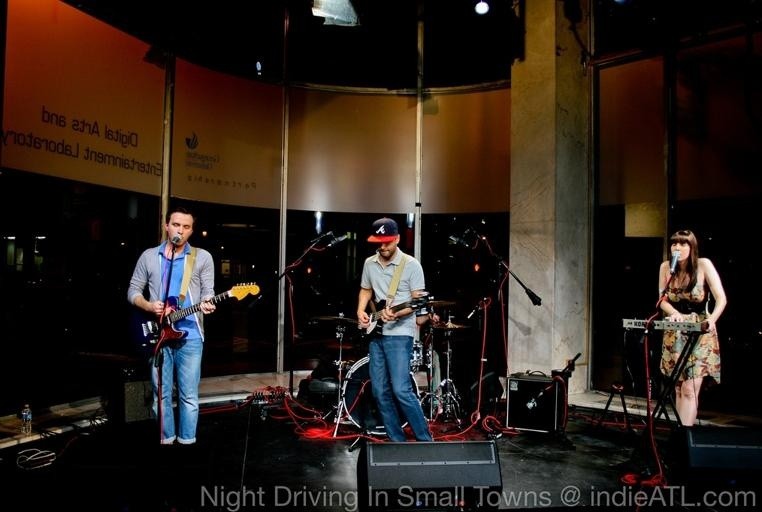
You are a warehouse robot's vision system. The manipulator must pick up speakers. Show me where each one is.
[506,375,569,433]
[357,440,503,512]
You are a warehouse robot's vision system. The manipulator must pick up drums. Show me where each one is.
[408,343,423,368]
[341,352,421,437]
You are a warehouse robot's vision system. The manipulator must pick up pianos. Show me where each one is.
[621,318,709,332]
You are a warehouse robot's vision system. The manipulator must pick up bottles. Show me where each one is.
[21,404,32,437]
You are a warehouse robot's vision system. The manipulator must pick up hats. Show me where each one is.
[368,218,398,243]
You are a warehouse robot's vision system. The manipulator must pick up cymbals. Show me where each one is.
[417,299,457,308]
[432,315,470,331]
[309,311,357,327]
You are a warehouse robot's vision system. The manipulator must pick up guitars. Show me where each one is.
[359,293,434,342]
[126,281,261,359]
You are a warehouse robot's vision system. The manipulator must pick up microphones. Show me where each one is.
[467,304,480,319]
[449,235,469,247]
[327,235,348,247]
[171,234,183,244]
[670,251,681,274]
[526,392,544,409]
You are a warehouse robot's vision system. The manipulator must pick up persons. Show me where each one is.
[126,203,219,446]
[413,310,444,415]
[232,302,306,373]
[655,229,729,430]
[352,216,433,443]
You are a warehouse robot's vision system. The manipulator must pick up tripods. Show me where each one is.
[432,329,460,422]
[420,306,460,424]
[434,350,462,404]
[321,332,345,420]
